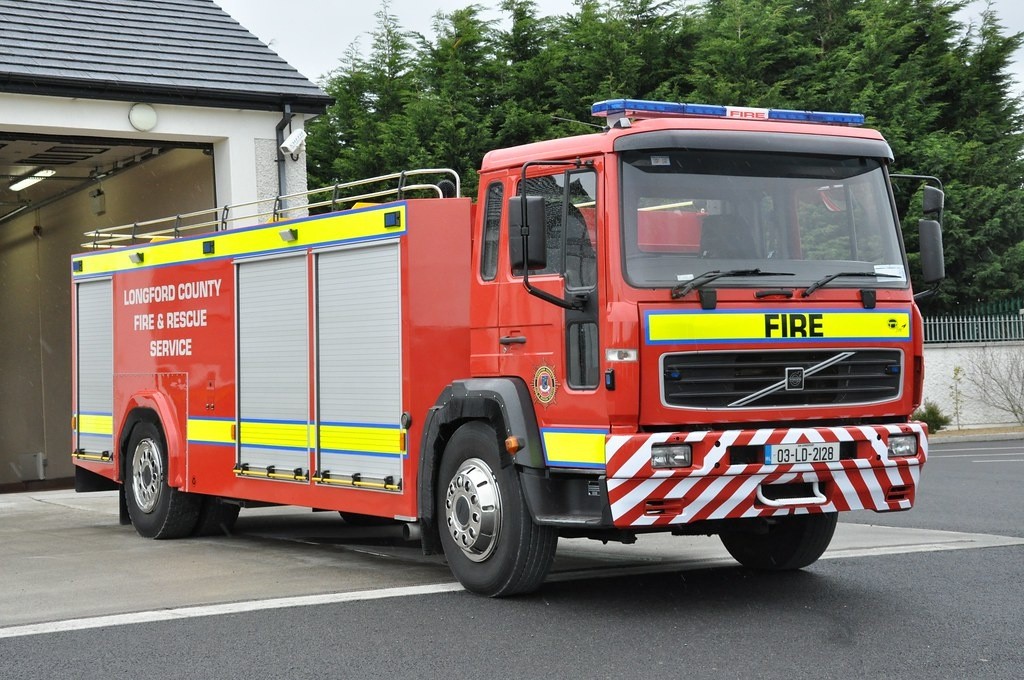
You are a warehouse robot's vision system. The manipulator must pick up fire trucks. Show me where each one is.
[69,97,947,597]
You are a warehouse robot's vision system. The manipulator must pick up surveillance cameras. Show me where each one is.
[279,128,307,155]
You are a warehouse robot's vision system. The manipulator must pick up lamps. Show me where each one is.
[89,189,104,214]
[9,167,56,191]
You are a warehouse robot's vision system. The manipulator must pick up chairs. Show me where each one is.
[699,213,759,259]
[545,202,596,289]
